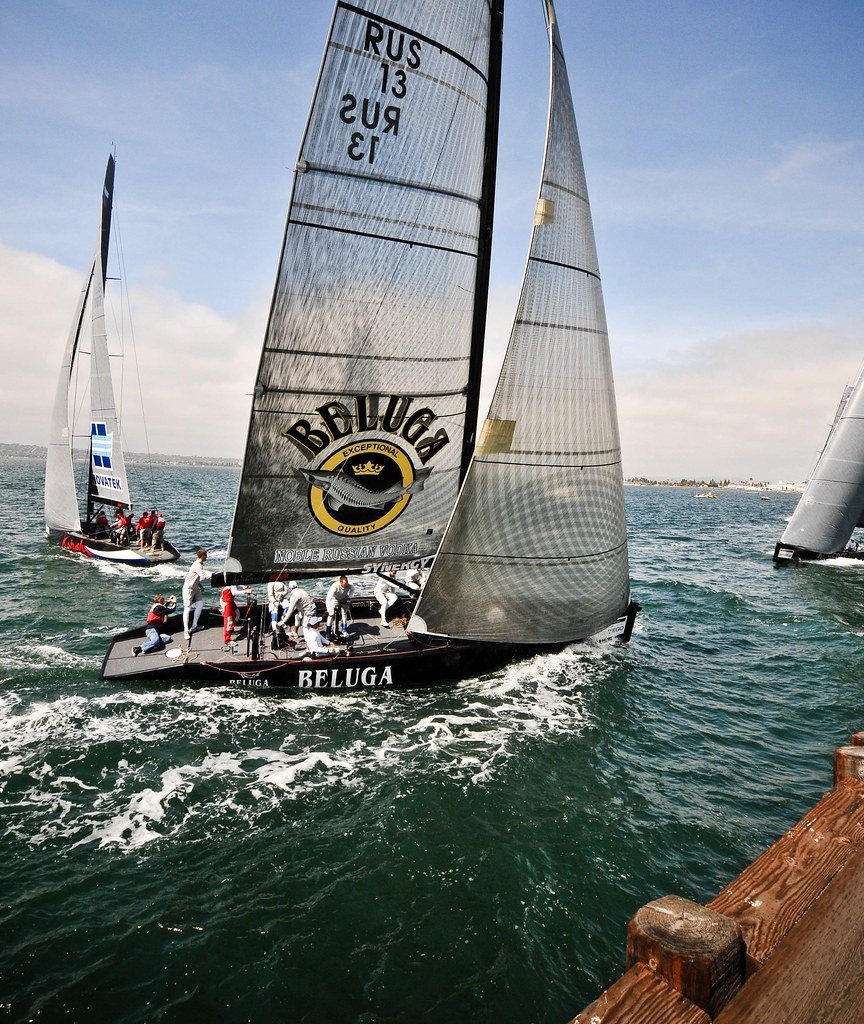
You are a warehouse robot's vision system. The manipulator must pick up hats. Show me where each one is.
[288,581,298,589]
[157,512,163,516]
[310,616,322,626]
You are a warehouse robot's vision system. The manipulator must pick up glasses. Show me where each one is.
[391,570,397,575]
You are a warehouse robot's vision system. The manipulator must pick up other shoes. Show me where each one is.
[342,631,349,637]
[379,608,385,615]
[325,633,331,641]
[136,540,165,553]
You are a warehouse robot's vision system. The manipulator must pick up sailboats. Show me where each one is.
[99,0,641,693]
[45,142,181,568]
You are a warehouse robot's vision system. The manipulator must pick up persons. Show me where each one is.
[305,616,339,653]
[406,569,427,598]
[374,571,398,627]
[182,550,214,639]
[220,585,252,646]
[131,594,176,657]
[94,507,165,552]
[278,582,316,656]
[326,576,355,639]
[268,582,289,630]
[846,538,856,551]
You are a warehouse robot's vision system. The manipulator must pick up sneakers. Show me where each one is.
[224,640,238,647]
[132,646,142,657]
[183,629,190,640]
[192,625,205,632]
[380,620,390,627]
[286,631,299,639]
